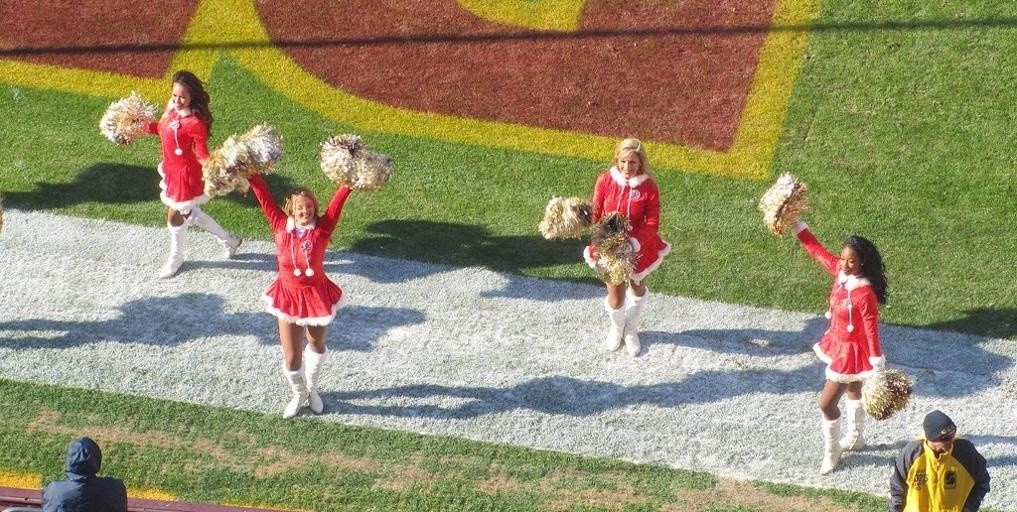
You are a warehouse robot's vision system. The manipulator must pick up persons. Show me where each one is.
[43,438,128,511]
[586,138,673,357]
[204,125,393,419]
[889,410,990,512]
[98,71,243,278]
[792,214,890,476]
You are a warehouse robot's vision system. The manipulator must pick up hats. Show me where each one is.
[923,410,956,442]
[66,439,102,476]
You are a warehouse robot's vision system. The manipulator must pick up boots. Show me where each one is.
[186,208,242,259]
[159,216,188,279]
[820,409,843,475]
[283,343,329,419]
[604,286,649,356]
[839,394,865,452]
